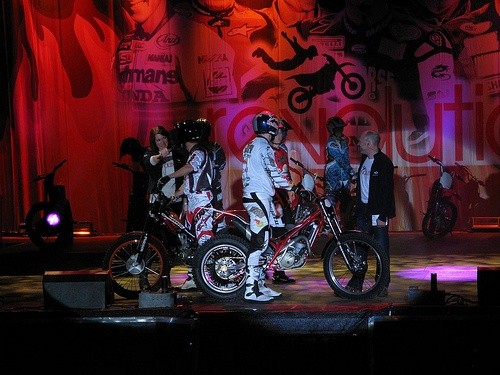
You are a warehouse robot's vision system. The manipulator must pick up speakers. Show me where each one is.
[43,270,115,310]
[477,267,500,303]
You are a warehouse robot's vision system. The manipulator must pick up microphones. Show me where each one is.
[157,145,177,160]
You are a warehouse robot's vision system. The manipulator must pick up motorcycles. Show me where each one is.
[421,154,465,240]
[271,156,325,236]
[103,176,247,299]
[27,159,74,247]
[191,188,390,304]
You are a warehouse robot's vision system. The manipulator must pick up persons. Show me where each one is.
[140,119,224,289]
[268,119,296,285]
[112,138,149,232]
[241,111,315,303]
[320,116,362,236]
[334,130,396,297]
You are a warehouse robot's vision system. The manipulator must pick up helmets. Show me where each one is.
[173,118,201,143]
[253,114,278,135]
[326,115,346,132]
[194,118,211,138]
[274,118,290,130]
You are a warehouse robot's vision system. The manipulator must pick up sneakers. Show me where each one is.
[178,271,196,289]
[245,286,283,303]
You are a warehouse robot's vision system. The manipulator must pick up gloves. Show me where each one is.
[156,175,169,189]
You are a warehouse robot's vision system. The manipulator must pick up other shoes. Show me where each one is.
[139,275,169,293]
[334,285,388,298]
